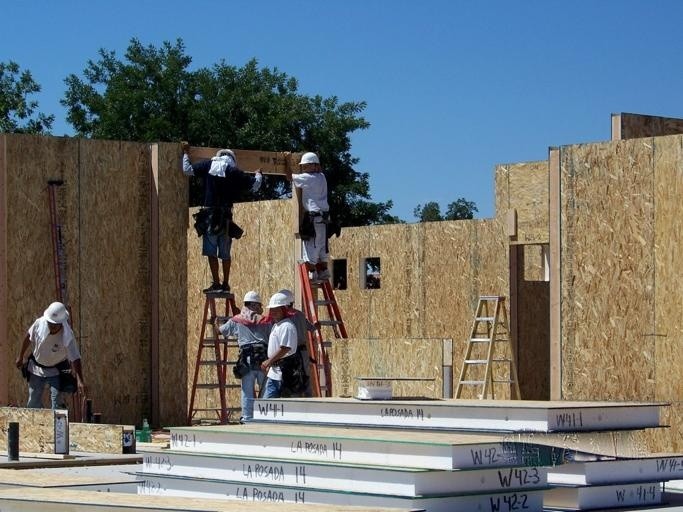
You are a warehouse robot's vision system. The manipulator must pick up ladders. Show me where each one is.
[187,290,258,427]
[455,296,522,399]
[297,260,348,397]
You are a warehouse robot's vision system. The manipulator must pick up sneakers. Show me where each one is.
[202,280,231,294]
[306,269,318,281]
[319,268,332,279]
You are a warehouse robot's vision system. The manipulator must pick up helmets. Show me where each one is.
[298,151,321,166]
[43,301,70,325]
[265,288,296,309]
[215,148,238,169]
[242,291,264,306]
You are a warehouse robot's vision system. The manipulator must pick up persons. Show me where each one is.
[263,292,298,399]
[180,141,263,293]
[282,150,331,283]
[16,301,88,410]
[210,291,271,421]
[278,289,318,397]
